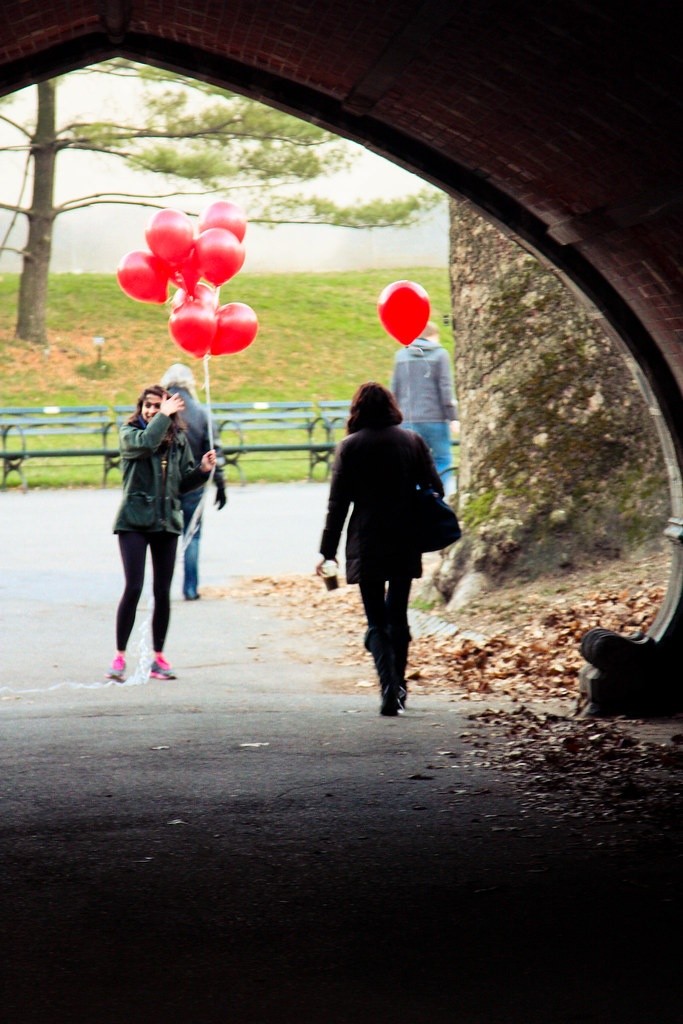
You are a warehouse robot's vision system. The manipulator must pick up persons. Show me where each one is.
[105,384,217,683]
[158,363,227,600]
[315,381,445,717]
[391,322,459,499]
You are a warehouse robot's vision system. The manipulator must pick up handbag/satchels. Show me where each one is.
[404,429,462,553]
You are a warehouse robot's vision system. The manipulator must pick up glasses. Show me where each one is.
[141,402,161,410]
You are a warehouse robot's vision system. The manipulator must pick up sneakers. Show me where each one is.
[150,656,176,678]
[105,655,127,683]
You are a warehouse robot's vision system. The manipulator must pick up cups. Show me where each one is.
[320,563,338,591]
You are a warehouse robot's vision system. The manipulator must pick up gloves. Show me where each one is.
[212,478,227,510]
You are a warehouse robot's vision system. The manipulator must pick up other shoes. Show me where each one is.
[185,594,201,601]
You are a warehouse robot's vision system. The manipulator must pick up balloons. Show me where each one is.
[117,250,169,304]
[167,239,201,286]
[209,303,258,356]
[169,303,217,361]
[198,201,246,243]
[196,228,244,286]
[172,282,221,311]
[145,208,192,262]
[378,280,431,347]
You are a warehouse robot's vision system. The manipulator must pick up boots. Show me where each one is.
[364,621,412,717]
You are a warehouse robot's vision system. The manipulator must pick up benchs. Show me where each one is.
[311,399,354,472]
[0,406,119,494]
[112,402,335,485]
